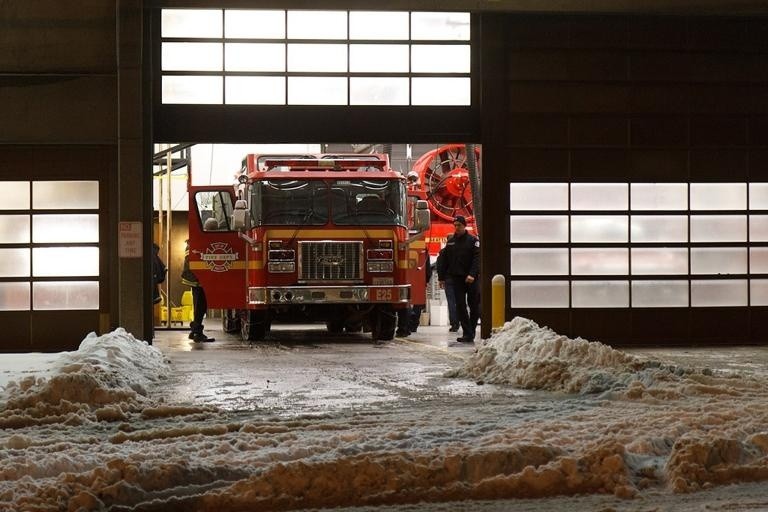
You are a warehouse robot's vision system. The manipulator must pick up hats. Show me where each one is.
[451,214,467,227]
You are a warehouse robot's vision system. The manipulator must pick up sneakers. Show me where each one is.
[448,324,475,343]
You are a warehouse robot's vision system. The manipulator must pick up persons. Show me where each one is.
[395,215,482,343]
[179,217,221,344]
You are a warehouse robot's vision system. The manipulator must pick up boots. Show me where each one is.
[189,321,216,343]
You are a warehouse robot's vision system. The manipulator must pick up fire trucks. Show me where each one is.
[185,150,433,349]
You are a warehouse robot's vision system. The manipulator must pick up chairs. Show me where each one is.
[265,188,388,221]
